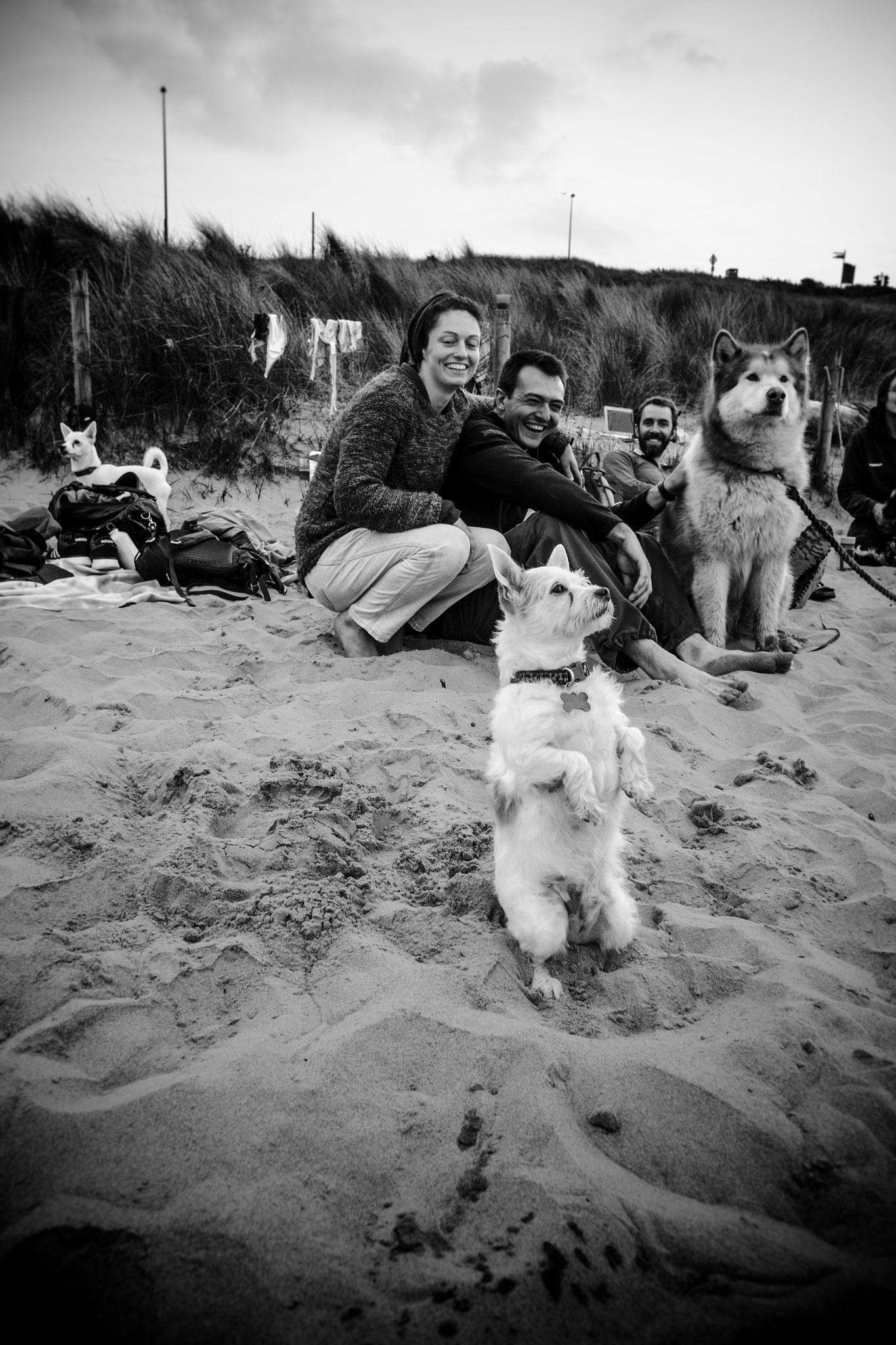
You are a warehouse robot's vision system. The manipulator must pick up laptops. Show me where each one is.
[603,405,634,439]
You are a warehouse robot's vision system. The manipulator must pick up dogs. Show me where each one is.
[59,421,172,533]
[660,327,810,655]
[486,546,658,999]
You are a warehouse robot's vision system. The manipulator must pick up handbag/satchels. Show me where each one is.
[48,472,167,548]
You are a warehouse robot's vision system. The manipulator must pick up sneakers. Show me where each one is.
[90,533,119,569]
[46,532,92,566]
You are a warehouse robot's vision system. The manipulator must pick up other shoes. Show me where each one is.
[791,519,833,582]
[793,557,828,609]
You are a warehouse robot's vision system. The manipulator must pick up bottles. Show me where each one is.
[109,528,141,569]
[837,536,856,571]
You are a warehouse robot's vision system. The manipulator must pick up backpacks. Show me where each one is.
[135,521,288,606]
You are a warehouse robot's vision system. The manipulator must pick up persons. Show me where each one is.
[838,369,896,566]
[412,351,794,710]
[294,293,586,659]
[603,396,687,544]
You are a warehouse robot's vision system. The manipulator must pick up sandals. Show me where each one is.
[809,582,836,600]
